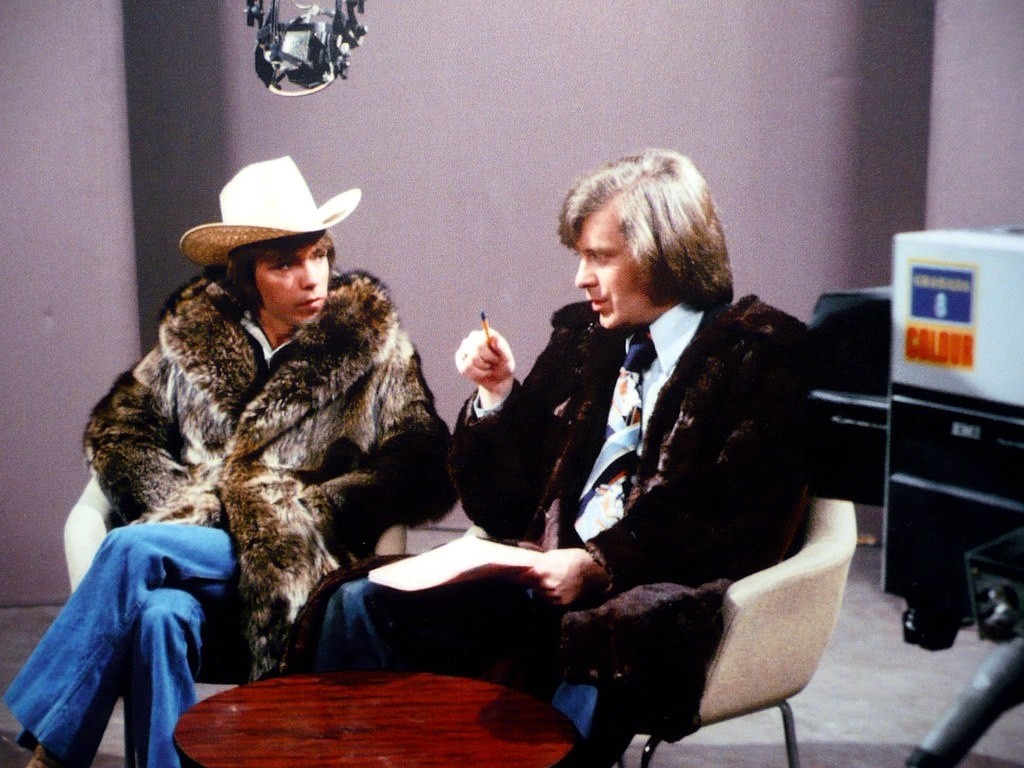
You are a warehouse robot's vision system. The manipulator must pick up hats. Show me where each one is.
[178,156,362,266]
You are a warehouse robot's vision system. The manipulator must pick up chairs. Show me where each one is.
[464,497,857,768]
[64,477,407,768]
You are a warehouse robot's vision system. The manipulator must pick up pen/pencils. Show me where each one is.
[480,312,492,344]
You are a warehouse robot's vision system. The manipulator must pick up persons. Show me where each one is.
[4,154,463,768]
[323,146,816,767]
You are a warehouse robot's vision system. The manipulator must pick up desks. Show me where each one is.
[172,672,576,768]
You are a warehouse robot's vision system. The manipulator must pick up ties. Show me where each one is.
[573,328,657,541]
